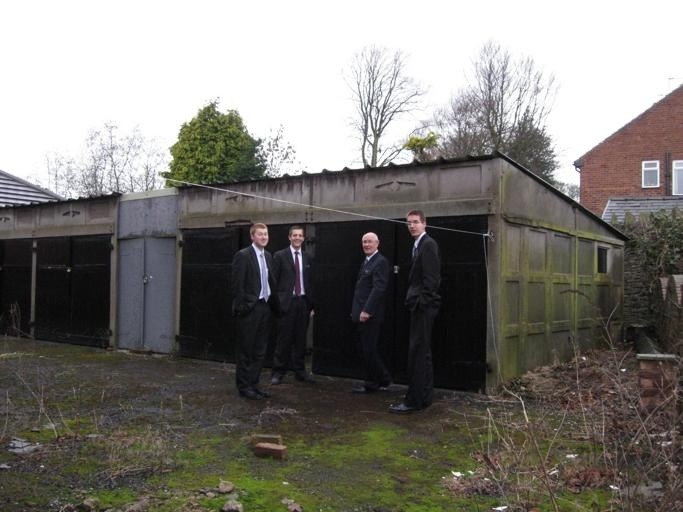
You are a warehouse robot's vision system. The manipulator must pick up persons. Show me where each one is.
[383,209,443,415]
[230,222,285,403]
[266,225,318,386]
[349,230,393,394]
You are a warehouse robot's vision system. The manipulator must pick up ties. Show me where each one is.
[259,253,269,303]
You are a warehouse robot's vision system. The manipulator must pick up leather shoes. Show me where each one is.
[253,385,271,398]
[350,382,431,414]
[239,387,258,399]
[271,371,284,386]
[294,251,301,297]
[295,370,312,382]
